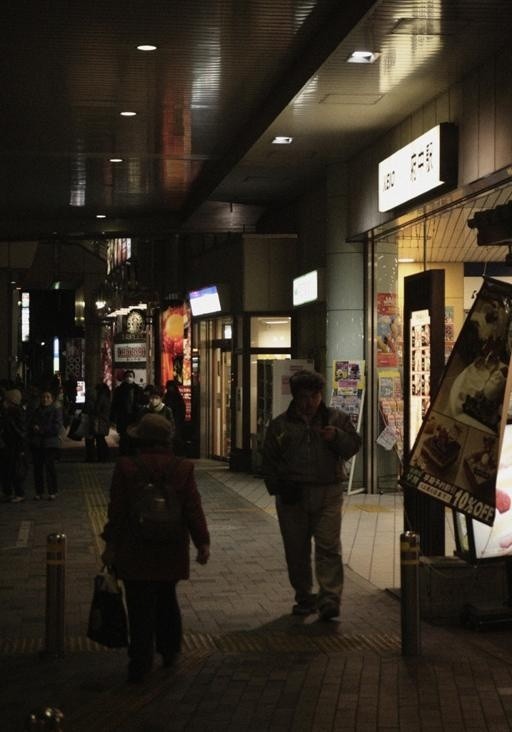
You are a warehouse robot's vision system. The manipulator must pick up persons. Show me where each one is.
[99,414,214,683]
[1,370,187,502]
[258,368,365,623]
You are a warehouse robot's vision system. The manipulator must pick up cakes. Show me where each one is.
[464,437,498,495]
[421,426,461,473]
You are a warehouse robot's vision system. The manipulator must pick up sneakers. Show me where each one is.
[319,605,339,620]
[292,602,317,614]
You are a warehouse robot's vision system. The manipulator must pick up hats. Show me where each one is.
[126,413,175,443]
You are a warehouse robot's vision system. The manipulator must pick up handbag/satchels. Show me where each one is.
[85,557,130,647]
